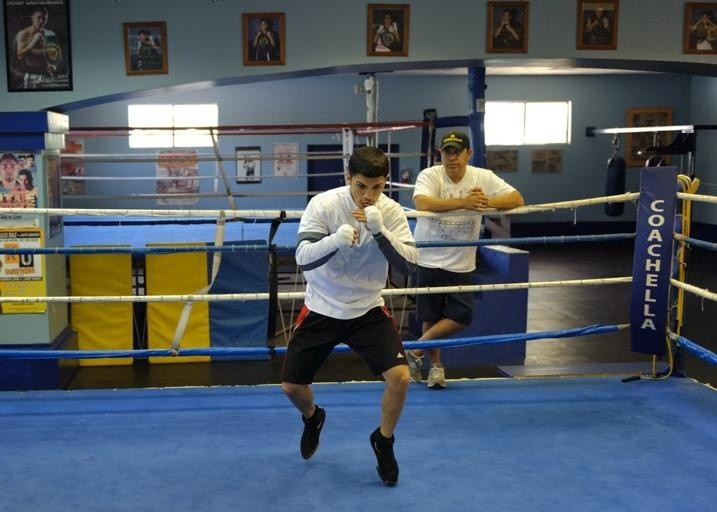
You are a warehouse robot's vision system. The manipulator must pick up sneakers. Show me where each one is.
[370,426,399,488]
[300,404,326,460]
[426,364,448,389]
[405,349,424,384]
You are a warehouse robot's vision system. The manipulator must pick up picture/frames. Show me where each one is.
[239,11,286,67]
[485,0,529,56]
[364,3,411,58]
[3,1,74,94]
[682,0,717,55]
[121,20,170,78]
[624,106,674,168]
[574,0,619,52]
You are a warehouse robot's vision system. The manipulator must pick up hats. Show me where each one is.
[440,130,470,153]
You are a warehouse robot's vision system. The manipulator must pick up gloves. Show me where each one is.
[364,205,383,234]
[335,223,358,250]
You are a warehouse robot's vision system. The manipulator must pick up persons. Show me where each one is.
[372,13,400,52]
[136,30,163,70]
[494,9,523,47]
[404,132,525,389]
[282,146,419,486]
[253,18,276,61]
[0,152,37,208]
[692,11,717,50]
[16,6,63,88]
[586,5,611,45]
